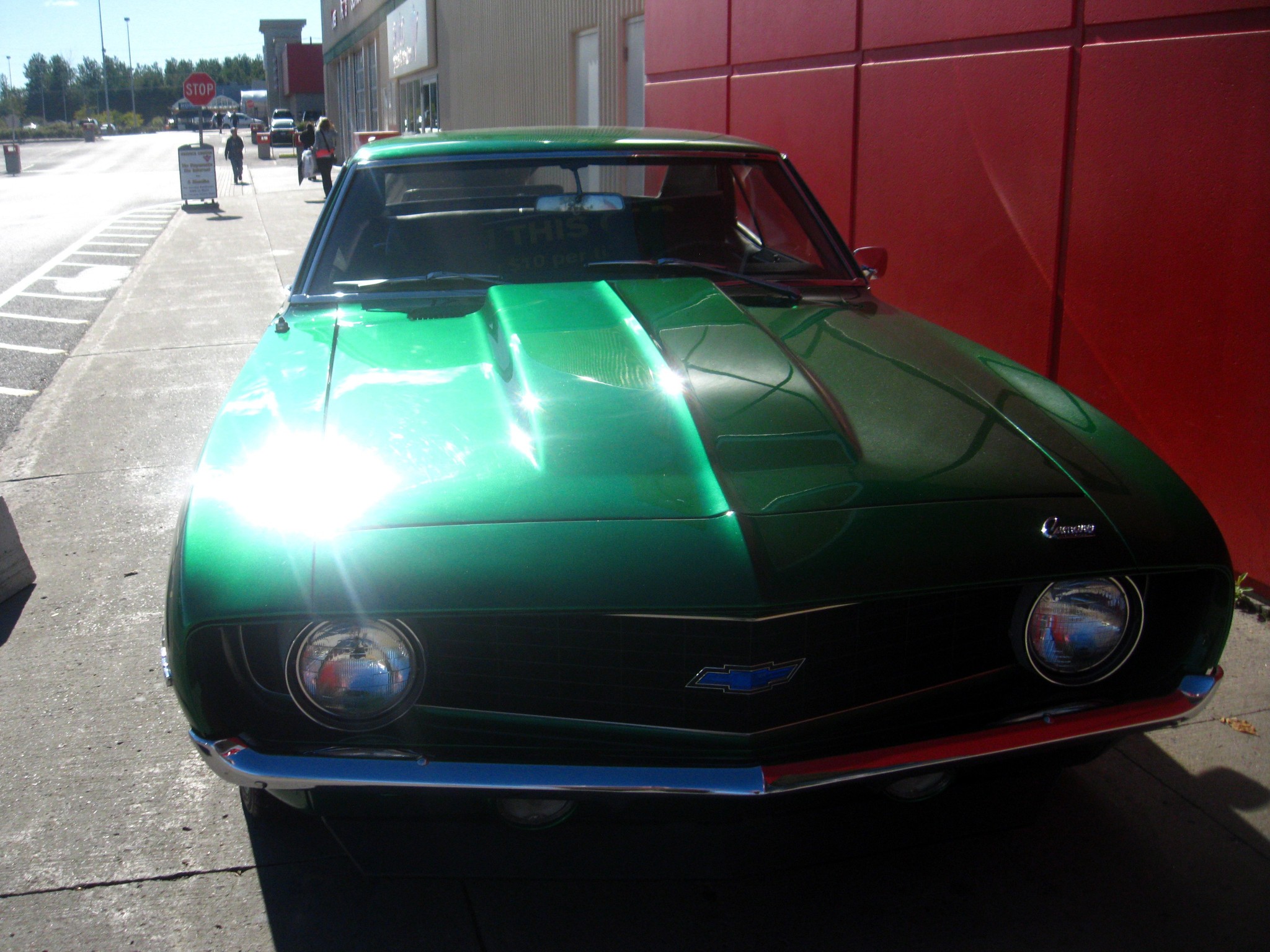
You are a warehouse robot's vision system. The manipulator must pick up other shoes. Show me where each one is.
[238,173,242,180]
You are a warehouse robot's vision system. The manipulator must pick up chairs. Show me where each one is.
[380,161,739,275]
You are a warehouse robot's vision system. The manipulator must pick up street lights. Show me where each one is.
[124,17,136,131]
[7,56,16,144]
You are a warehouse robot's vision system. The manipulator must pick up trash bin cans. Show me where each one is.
[257,133,270,158]
[250,124,261,145]
[295,133,305,166]
[3,143,21,174]
[83,123,95,142]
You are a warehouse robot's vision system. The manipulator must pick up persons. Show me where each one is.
[215,109,224,134]
[230,109,239,128]
[299,116,338,201]
[224,127,244,185]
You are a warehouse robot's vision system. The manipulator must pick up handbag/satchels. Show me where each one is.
[332,154,338,164]
[302,150,321,178]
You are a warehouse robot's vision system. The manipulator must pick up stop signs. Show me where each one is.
[182,71,216,106]
[245,100,254,108]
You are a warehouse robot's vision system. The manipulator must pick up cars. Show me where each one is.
[269,119,297,143]
[151,129,1229,874]
[100,123,114,131]
[270,108,294,122]
[218,113,265,128]
[79,119,98,129]
[302,110,318,124]
